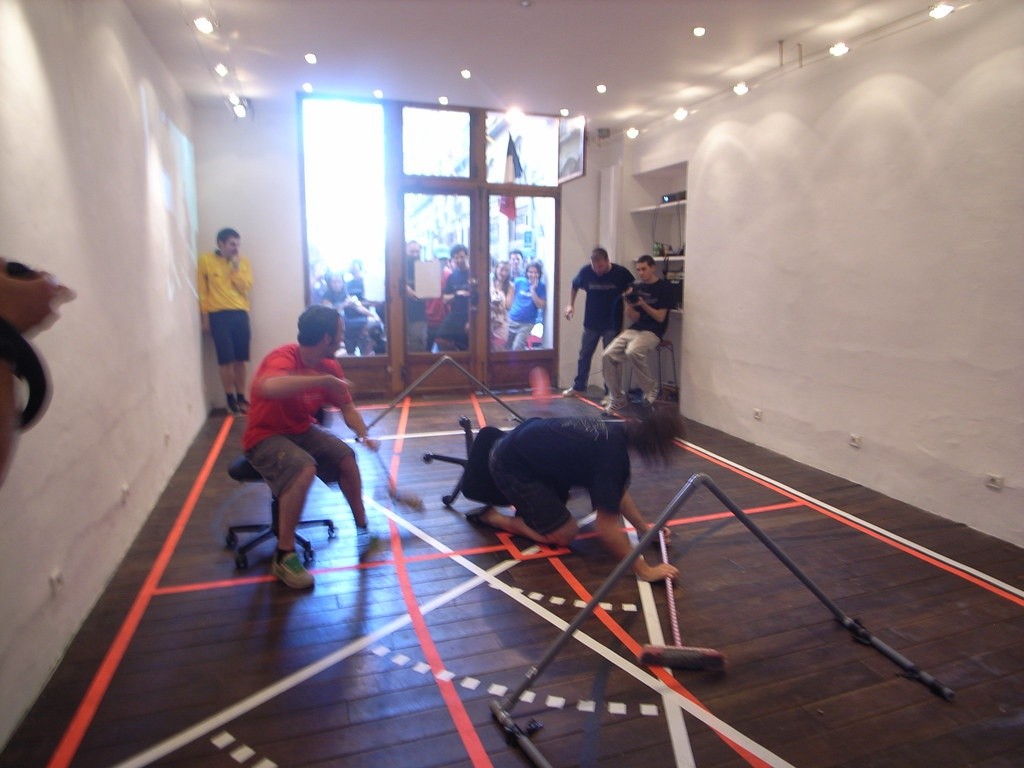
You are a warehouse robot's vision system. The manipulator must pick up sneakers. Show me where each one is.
[563,386,587,396]
[600,395,608,404]
[642,388,659,404]
[602,392,629,416]
[271,550,315,588]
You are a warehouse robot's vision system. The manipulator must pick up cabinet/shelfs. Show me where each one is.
[631,199,686,313]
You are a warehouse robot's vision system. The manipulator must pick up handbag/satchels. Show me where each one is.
[414,259,442,299]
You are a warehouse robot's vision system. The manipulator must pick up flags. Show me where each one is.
[498,138,522,221]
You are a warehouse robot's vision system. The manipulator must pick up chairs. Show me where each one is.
[226,410,338,571]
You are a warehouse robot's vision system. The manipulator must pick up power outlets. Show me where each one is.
[849,433,861,449]
[753,408,762,420]
[985,473,1004,489]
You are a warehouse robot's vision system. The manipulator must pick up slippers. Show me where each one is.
[464,505,491,524]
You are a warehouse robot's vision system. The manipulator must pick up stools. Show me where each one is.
[629,340,678,400]
[421,414,513,508]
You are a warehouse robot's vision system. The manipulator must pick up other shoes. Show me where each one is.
[238,396,251,413]
[227,396,242,414]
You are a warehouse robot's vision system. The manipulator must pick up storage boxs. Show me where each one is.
[413,259,442,299]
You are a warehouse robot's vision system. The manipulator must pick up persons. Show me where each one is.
[505,262,546,350]
[465,413,683,582]
[562,249,637,404]
[241,305,380,591]
[313,241,543,356]
[198,229,252,416]
[0,259,76,482]
[600,255,673,416]
